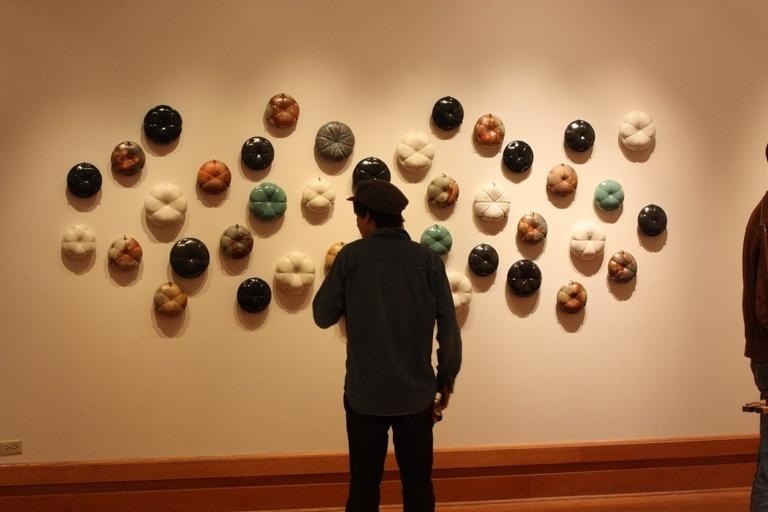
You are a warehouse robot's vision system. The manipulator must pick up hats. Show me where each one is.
[344,179,408,209]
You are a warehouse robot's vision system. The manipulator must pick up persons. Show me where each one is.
[743,144,768,511]
[311,181,463,511]
